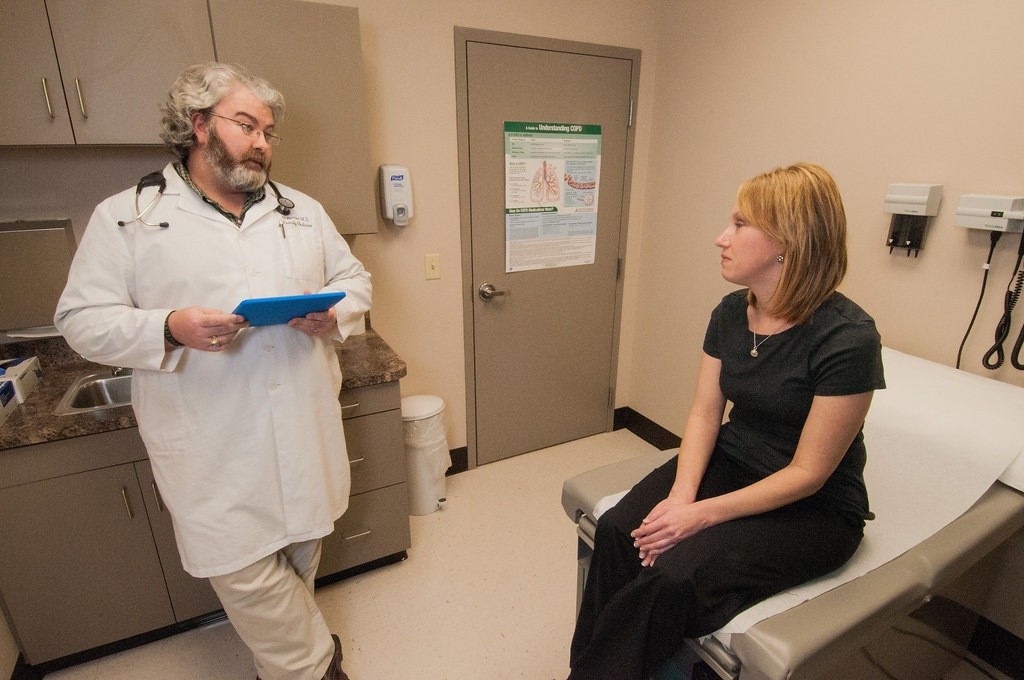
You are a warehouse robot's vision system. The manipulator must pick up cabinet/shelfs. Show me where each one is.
[0,0,379,235]
[0,376,411,680]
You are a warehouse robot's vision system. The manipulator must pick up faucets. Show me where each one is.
[111,365,121,375]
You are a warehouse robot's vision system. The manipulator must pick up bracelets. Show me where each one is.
[164,311,183,346]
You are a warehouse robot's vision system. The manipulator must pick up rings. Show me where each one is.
[313,329,317,333]
[209,336,220,351]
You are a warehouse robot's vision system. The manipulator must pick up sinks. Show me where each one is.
[51,369,132,417]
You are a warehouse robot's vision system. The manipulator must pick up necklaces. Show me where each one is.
[750,306,786,358]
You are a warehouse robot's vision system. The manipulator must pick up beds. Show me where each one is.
[560,345,1024,680]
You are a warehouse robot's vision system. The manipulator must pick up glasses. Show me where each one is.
[204,111,283,146]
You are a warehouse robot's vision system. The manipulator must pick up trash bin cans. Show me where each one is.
[401,394,452,517]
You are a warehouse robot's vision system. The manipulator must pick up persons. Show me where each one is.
[53,61,374,680]
[569,162,887,680]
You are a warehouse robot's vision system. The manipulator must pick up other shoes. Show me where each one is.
[322,634,349,680]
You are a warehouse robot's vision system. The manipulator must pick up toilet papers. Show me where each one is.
[349,307,367,337]
